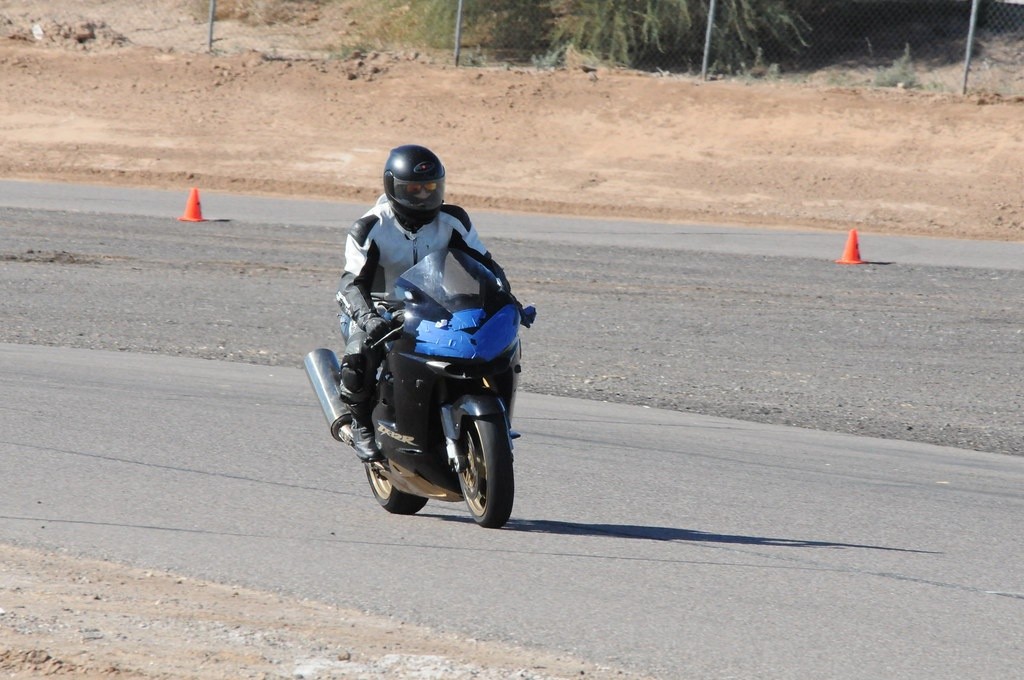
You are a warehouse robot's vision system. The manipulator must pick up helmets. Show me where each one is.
[382,145,445,224]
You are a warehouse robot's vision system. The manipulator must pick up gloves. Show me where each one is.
[364,318,391,340]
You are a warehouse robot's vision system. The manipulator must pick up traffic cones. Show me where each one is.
[177,188,209,223]
[835,229,868,265]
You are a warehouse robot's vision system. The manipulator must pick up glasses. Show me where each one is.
[404,182,437,193]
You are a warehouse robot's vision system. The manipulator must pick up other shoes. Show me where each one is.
[350,418,383,461]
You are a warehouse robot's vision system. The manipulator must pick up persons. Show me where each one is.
[337,145,521,461]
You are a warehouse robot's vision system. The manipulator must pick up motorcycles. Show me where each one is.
[303,247,537,530]
